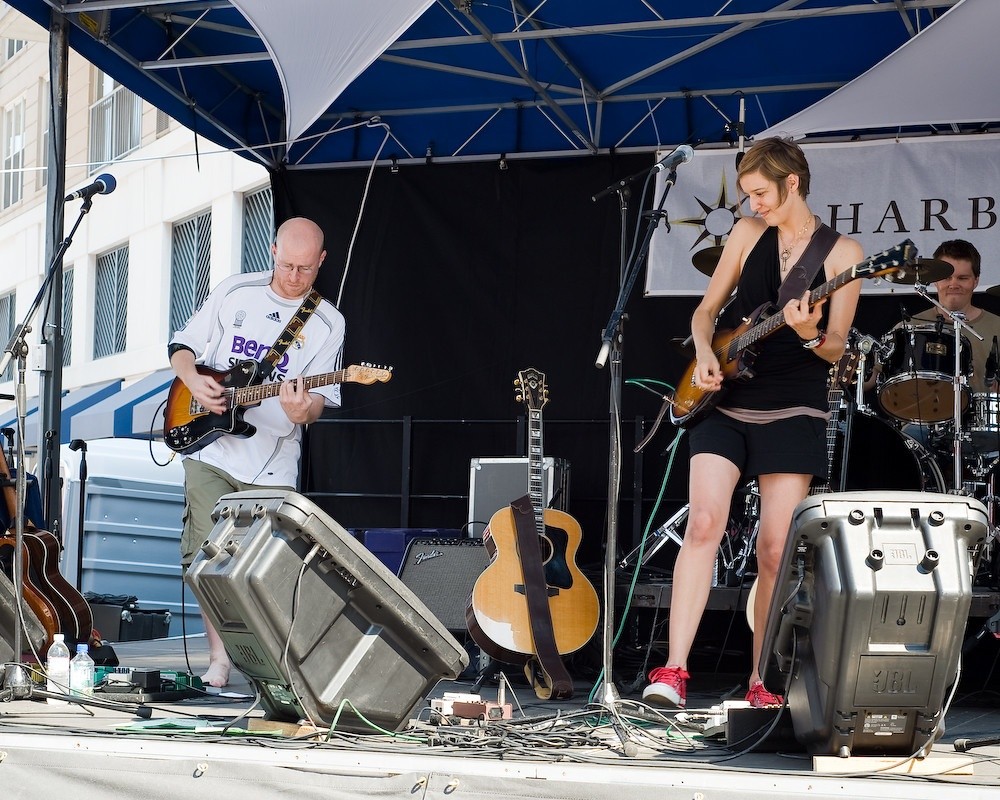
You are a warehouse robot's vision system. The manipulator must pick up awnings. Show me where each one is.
[1,378,126,451]
[69,367,180,444]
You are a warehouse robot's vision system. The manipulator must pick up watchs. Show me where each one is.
[799,329,821,350]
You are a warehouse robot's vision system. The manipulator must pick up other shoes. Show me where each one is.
[744,680,788,708]
[642,666,690,709]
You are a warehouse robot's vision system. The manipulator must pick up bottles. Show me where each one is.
[47,634,70,707]
[711,552,718,587]
[69,644,95,704]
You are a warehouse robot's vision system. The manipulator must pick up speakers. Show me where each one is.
[758,490,991,758]
[185,490,469,735]
[396,537,490,636]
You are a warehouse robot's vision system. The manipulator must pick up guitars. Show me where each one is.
[464,367,601,665]
[0,434,93,657]
[746,348,862,637]
[162,356,395,455]
[667,237,919,428]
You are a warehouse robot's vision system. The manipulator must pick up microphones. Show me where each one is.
[735,98,746,173]
[65,173,115,202]
[650,144,695,174]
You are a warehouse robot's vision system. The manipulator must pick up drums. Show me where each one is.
[878,323,974,425]
[825,404,946,493]
[928,391,1000,459]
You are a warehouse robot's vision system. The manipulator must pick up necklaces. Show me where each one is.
[777,208,814,273]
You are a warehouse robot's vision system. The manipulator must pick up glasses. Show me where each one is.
[273,253,320,274]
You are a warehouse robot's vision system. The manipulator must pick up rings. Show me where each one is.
[690,376,696,385]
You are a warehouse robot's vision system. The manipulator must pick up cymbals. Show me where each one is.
[691,245,725,277]
[881,258,954,285]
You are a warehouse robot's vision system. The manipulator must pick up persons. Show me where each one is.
[165,216,346,690]
[640,135,861,713]
[868,238,1000,492]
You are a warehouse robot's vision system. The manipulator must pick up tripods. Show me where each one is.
[1,200,154,721]
[491,173,704,757]
